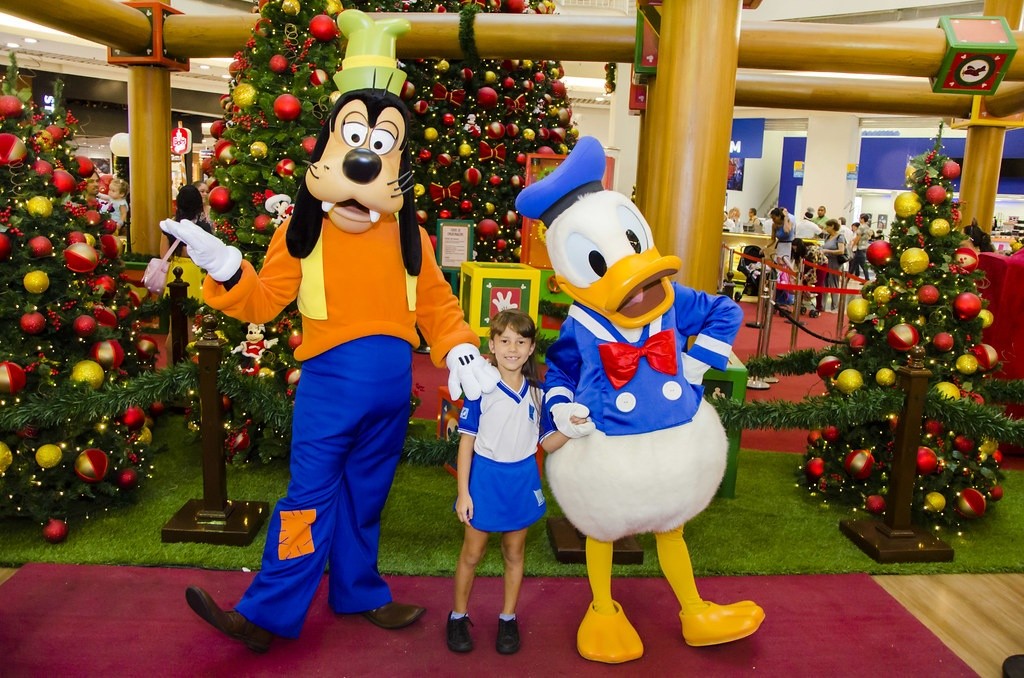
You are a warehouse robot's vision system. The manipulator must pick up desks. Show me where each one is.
[721,230,823,283]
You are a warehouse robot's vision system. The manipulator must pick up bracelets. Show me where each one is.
[118,220,124,226]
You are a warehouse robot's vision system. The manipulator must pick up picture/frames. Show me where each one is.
[520,153,615,269]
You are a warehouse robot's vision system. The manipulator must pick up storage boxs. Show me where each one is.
[435,262,577,479]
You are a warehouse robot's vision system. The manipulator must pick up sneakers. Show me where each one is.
[496,617,520,654]
[447,610,474,652]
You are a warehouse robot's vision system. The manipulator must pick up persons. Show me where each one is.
[796,212,827,238]
[83,170,130,251]
[161,185,212,258]
[445,308,588,653]
[172,180,183,211]
[790,238,829,315]
[724,206,883,313]
[190,181,212,221]
[770,209,794,262]
[846,213,872,284]
[747,208,761,225]
[818,219,847,311]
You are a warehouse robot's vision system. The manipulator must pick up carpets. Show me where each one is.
[0,416,1024,576]
[0,560,981,678]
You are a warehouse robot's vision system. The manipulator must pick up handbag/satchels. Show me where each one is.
[836,234,856,264]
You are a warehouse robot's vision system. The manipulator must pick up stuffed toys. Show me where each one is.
[263,189,294,225]
[531,99,546,119]
[159,10,503,652]
[231,324,279,372]
[515,134,766,664]
[464,113,481,137]
